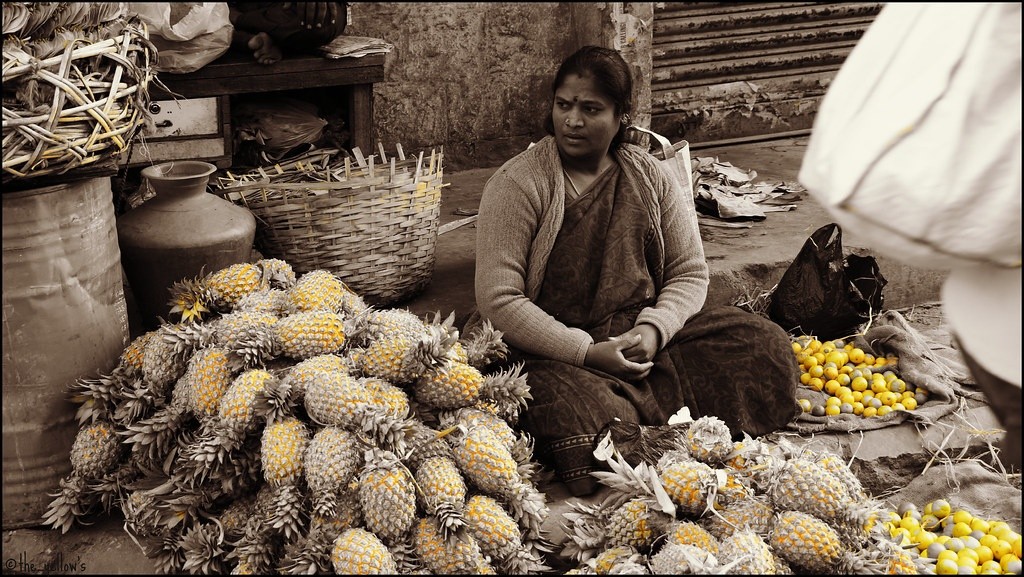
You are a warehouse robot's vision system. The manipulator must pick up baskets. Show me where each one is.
[2,2,187,187]
[216,141,453,305]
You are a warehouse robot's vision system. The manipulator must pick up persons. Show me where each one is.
[457,46,800,496]
[936,264,1024,475]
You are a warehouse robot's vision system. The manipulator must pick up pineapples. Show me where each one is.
[41,259,938,577]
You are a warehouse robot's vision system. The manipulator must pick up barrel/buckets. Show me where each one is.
[2,161,136,526]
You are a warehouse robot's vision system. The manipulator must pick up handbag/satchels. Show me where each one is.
[769,222,887,338]
[626,125,705,254]
[795,4,1023,276]
